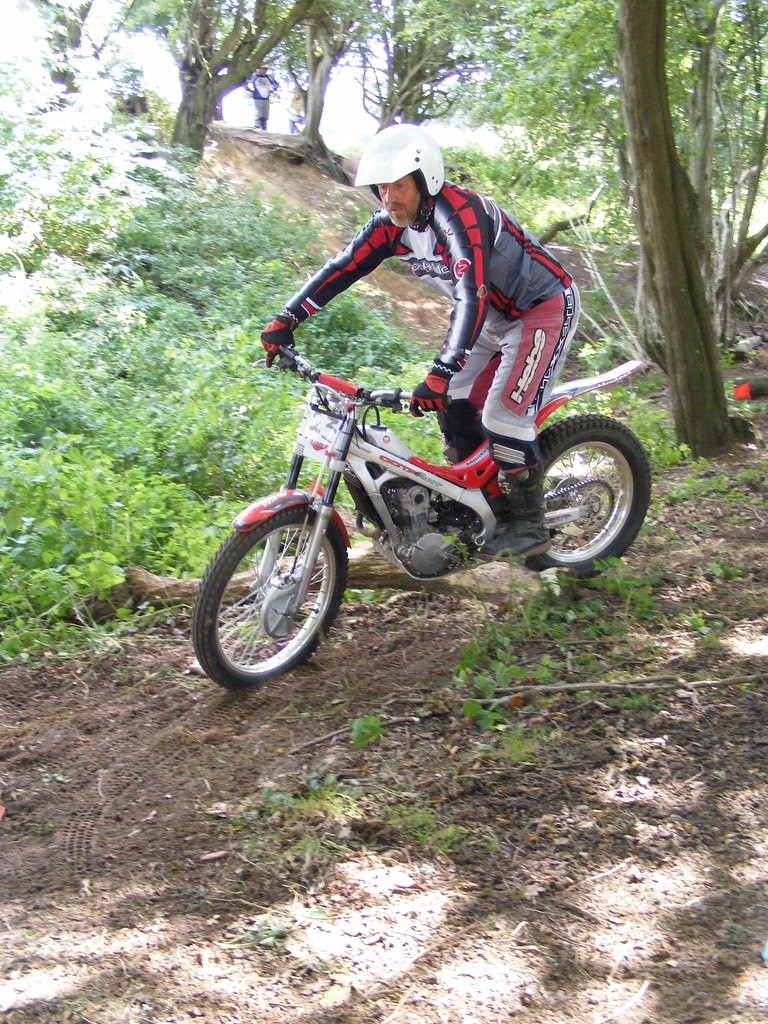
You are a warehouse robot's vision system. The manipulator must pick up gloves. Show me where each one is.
[410,369,452,417]
[261,314,295,368]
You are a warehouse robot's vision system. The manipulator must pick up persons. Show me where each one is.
[243,66,279,131]
[261,122,581,563]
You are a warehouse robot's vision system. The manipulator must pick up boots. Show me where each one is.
[480,464,551,562]
[255,118,268,130]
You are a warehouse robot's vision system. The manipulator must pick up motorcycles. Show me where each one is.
[183,340,657,696]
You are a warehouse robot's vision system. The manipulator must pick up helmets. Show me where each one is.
[355,123,445,196]
